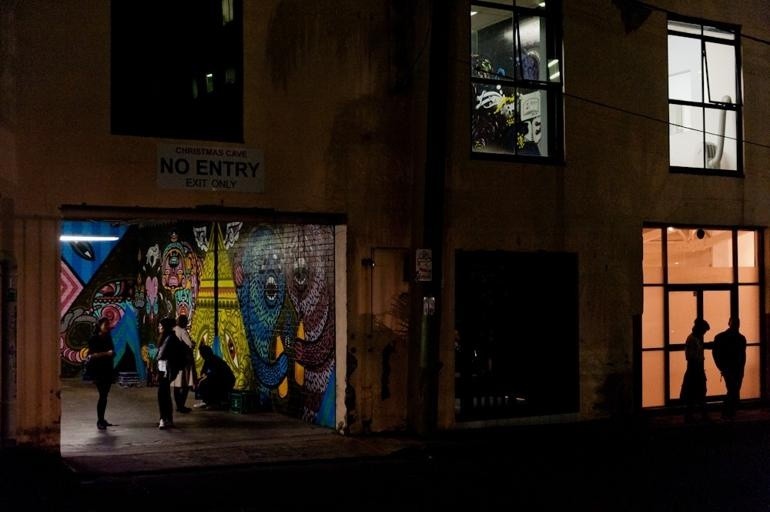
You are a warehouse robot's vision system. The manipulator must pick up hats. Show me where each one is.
[162,318,175,328]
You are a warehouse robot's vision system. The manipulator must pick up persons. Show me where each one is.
[678,318,710,406]
[713,316,746,418]
[87,317,116,430]
[156,319,179,430]
[199,345,235,410]
[172,315,196,413]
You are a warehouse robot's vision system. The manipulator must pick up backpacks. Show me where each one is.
[171,345,188,369]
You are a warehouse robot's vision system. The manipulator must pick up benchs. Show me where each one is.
[195,386,258,414]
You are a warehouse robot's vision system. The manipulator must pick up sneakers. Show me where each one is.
[97,421,106,430]
[104,421,112,426]
[159,419,166,429]
[177,407,192,413]
[165,417,174,427]
[193,402,207,408]
